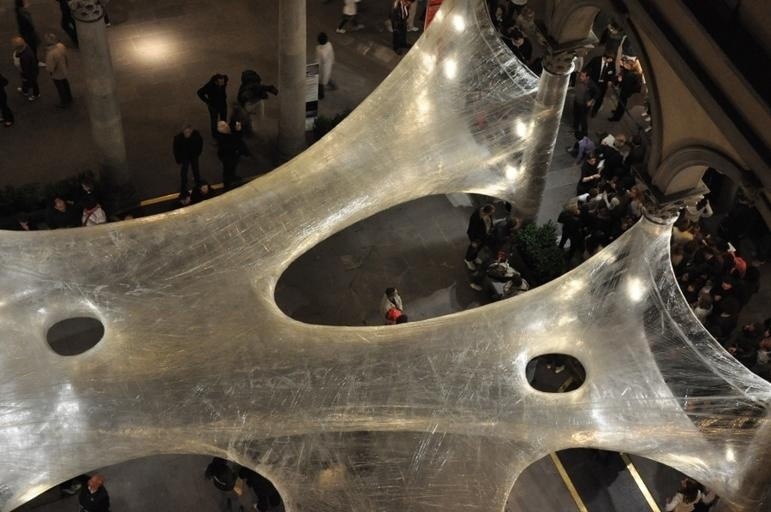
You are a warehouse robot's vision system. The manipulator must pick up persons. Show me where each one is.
[14,176,135,231]
[670,207,771,382]
[313,31,339,100]
[76,473,110,512]
[462,200,529,301]
[693,486,719,512]
[486,0,544,75]
[0,0,111,128]
[203,457,284,512]
[664,478,701,512]
[555,20,652,266]
[379,287,402,325]
[390,0,425,56]
[170,69,278,209]
[335,0,366,34]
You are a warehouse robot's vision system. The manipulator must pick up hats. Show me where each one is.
[11,36,24,45]
[44,33,57,44]
[217,121,228,134]
